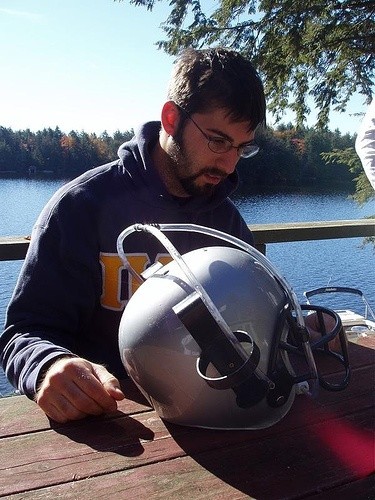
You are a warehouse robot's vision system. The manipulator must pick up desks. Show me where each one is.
[0,335,375,500]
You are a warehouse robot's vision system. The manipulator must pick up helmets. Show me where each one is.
[115,222,347,433]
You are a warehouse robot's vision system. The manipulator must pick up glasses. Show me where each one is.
[178,107,260,159]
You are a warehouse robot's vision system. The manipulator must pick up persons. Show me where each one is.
[1,46,348,423]
